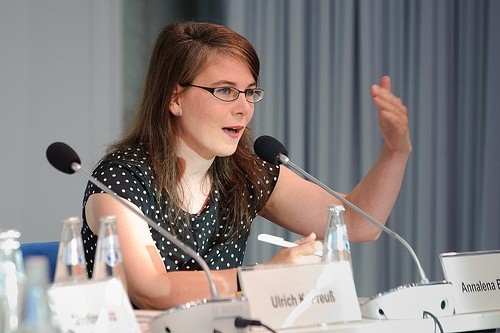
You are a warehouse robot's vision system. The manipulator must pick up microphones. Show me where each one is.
[46,142,254,332]
[254,135,454,320]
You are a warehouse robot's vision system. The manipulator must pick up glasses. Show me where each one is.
[179,84,265,103]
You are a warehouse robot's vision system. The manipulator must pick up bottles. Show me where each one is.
[321,205,353,266]
[91,215,129,298]
[53,217,88,285]
[0,230,33,333]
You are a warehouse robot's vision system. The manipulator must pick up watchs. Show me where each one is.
[237,265,252,292]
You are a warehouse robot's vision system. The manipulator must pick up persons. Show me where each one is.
[81,21,412,310]
